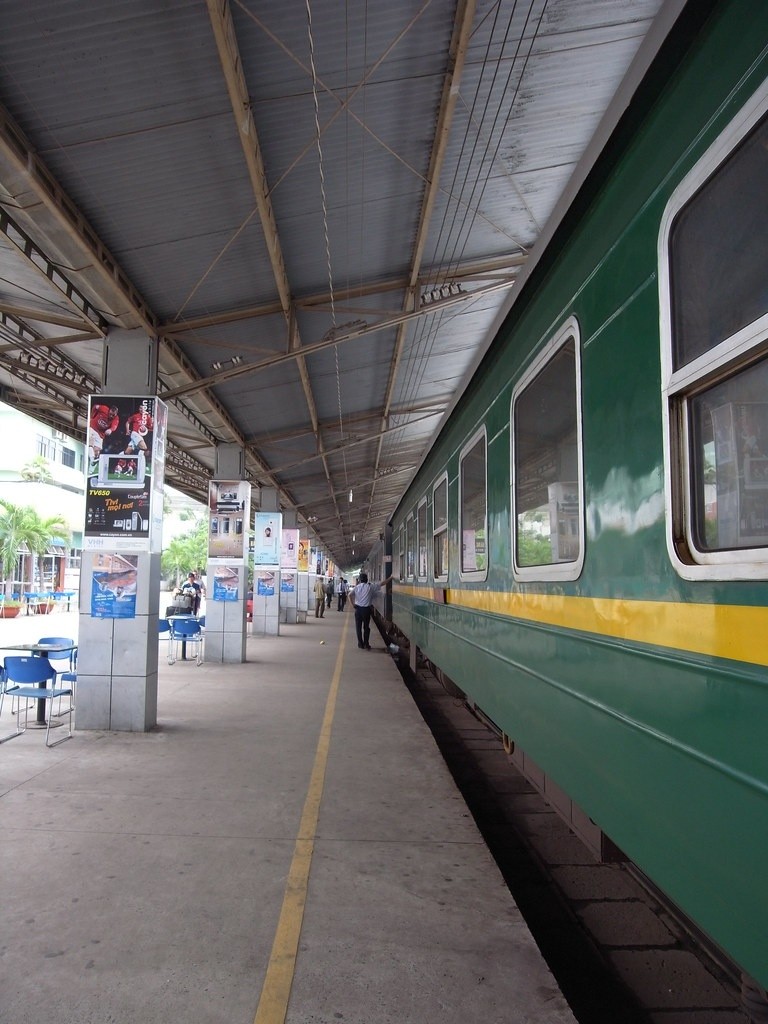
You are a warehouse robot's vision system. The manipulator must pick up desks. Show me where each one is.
[166,615,198,661]
[0,643,78,729]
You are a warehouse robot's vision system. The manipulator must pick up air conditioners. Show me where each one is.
[59,433,68,443]
[52,429,62,439]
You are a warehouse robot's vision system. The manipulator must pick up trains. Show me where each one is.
[360,0,768,1024]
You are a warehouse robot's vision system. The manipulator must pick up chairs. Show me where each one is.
[159,614,205,667]
[0,656,73,748]
[11,637,78,718]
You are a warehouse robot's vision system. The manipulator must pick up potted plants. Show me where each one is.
[28,598,60,614]
[0,599,25,618]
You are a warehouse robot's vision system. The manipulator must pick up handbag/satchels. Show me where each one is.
[166,606,194,617]
[172,593,191,608]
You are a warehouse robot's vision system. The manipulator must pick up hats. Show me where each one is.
[187,573,194,577]
[359,574,367,580]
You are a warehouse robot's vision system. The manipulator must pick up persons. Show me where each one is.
[326,577,359,612]
[119,404,154,455]
[348,573,392,649]
[314,577,328,618]
[54,585,61,600]
[89,405,119,474]
[115,458,136,478]
[265,528,271,537]
[249,586,253,617]
[181,571,206,616]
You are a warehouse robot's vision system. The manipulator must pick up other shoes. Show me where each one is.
[358,642,364,648]
[364,642,371,649]
